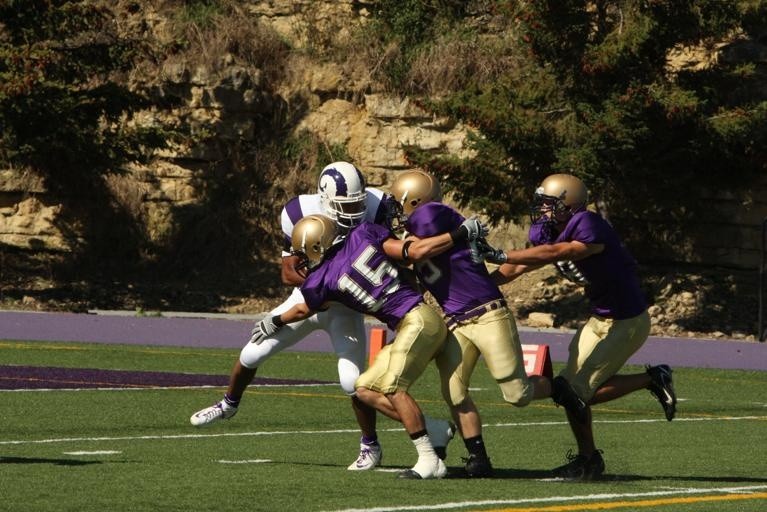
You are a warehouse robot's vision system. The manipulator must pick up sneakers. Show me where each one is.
[428,417,456,462]
[645,364,678,422]
[346,433,383,470]
[541,450,605,481]
[449,458,498,479]
[189,393,241,426]
[550,375,592,426]
[397,454,447,480]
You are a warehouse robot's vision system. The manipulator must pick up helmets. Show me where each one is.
[290,214,345,278]
[390,170,442,230]
[317,161,367,231]
[529,172,588,225]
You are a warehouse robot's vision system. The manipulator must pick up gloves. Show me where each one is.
[250,314,286,345]
[469,238,508,265]
[459,214,492,240]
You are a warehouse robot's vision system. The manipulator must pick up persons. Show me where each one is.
[475,173,678,483]
[387,171,585,481]
[251,213,490,481]
[189,161,397,476]
